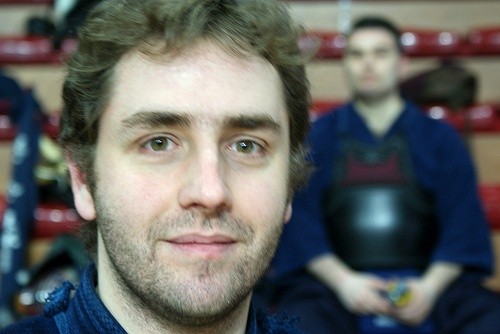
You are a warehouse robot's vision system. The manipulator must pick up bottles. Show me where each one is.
[385,282,416,306]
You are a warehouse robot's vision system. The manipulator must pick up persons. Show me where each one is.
[0,0,313,334]
[262,15,500,334]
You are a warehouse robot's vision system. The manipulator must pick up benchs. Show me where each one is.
[0,28,500,316]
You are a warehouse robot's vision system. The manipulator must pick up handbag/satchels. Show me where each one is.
[399,65,479,111]
